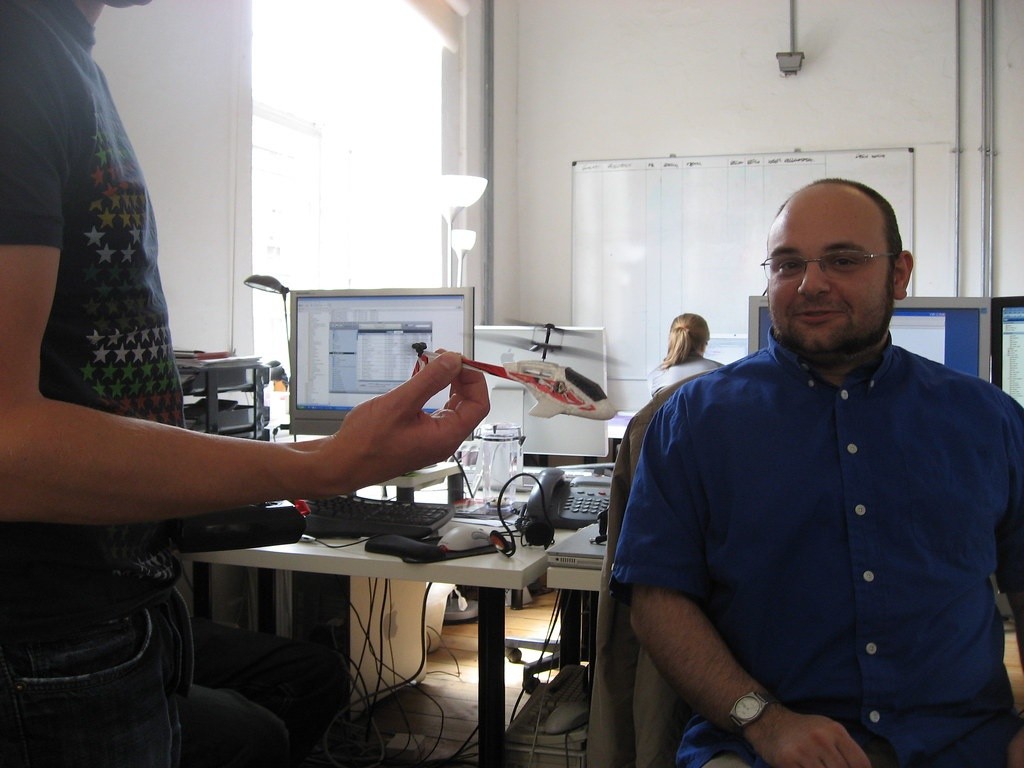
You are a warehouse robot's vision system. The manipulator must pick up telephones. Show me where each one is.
[526,467,615,530]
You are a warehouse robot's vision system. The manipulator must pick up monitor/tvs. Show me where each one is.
[286,286,477,443]
[746,295,1024,412]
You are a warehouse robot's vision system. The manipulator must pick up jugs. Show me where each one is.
[482,426,526,487]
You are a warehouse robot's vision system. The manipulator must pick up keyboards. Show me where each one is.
[266,494,455,539]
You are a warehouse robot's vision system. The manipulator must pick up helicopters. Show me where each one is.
[409,318,622,421]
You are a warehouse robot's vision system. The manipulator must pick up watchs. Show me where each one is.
[730,693,778,736]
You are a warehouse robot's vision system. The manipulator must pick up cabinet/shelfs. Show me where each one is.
[185,364,275,441]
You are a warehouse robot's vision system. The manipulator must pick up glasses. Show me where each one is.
[761,250,898,282]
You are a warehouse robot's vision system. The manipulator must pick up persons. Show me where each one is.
[610,178,1024,768]
[0,0,491,768]
[651,312,726,397]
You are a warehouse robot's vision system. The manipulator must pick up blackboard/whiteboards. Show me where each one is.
[571,147,915,381]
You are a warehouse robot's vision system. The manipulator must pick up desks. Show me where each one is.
[179,465,576,768]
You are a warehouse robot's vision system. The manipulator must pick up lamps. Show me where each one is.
[452,223,478,289]
[442,173,488,288]
[775,52,805,74]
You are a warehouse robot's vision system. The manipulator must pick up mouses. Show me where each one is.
[438,527,492,552]
